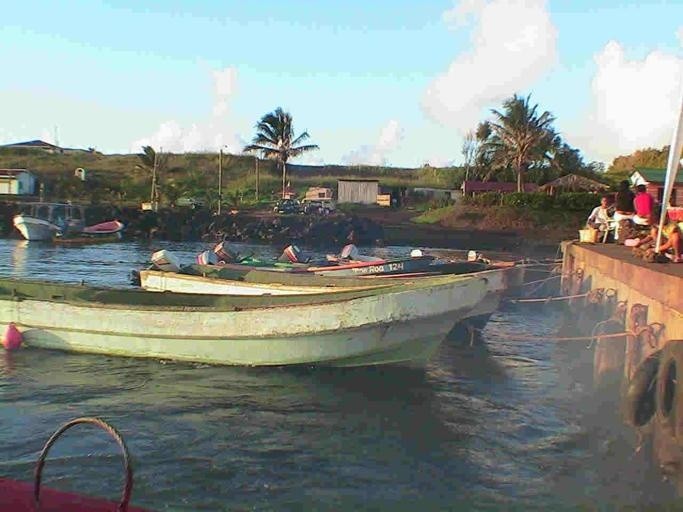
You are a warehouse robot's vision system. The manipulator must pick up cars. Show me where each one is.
[274,200,302,214]
[169,196,205,210]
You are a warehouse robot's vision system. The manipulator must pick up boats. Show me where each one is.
[12,213,126,242]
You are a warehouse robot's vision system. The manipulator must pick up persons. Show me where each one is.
[587,196,611,242]
[634,203,683,263]
[613,180,636,240]
[632,184,654,226]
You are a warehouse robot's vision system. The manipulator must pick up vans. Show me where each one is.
[301,198,335,213]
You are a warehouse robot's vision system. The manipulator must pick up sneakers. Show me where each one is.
[673,255,682,263]
